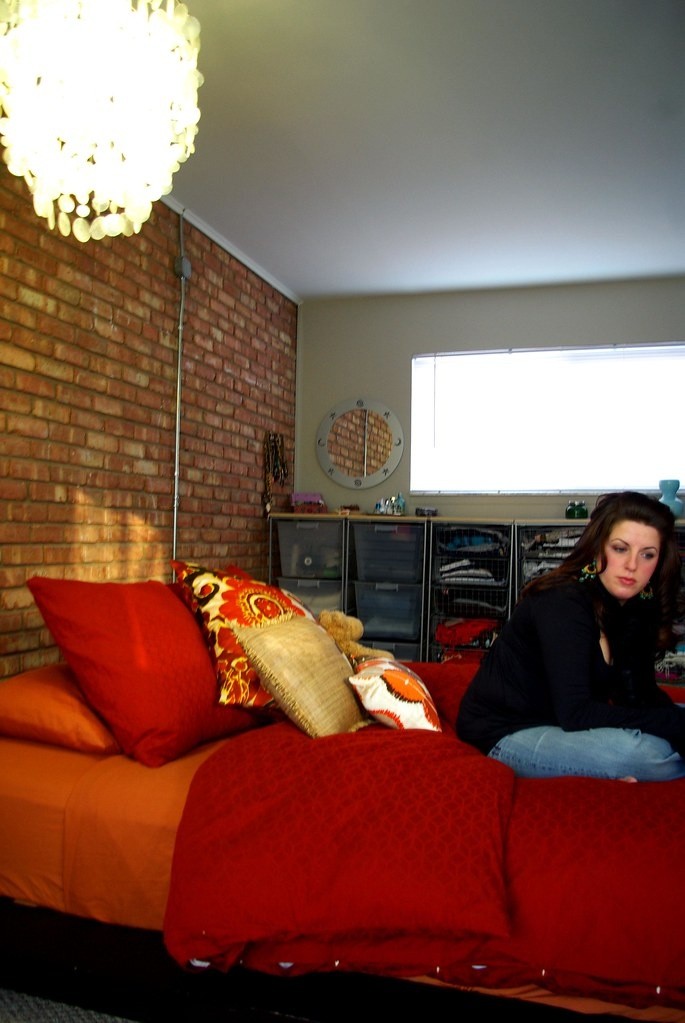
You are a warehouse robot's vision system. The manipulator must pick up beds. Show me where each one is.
[0,660,685,1023]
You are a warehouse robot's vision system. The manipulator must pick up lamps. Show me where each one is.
[0,0,205,243]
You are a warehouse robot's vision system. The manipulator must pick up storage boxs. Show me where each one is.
[275,578,343,618]
[353,581,420,643]
[358,640,420,663]
[277,520,344,578]
[351,523,423,582]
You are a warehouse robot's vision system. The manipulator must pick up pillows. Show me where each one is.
[0,662,118,755]
[233,612,366,736]
[347,655,443,733]
[25,564,285,767]
[169,560,311,714]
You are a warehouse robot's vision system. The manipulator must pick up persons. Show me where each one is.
[456,492,685,782]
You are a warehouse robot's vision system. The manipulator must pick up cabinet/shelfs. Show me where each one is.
[268,512,685,664]
